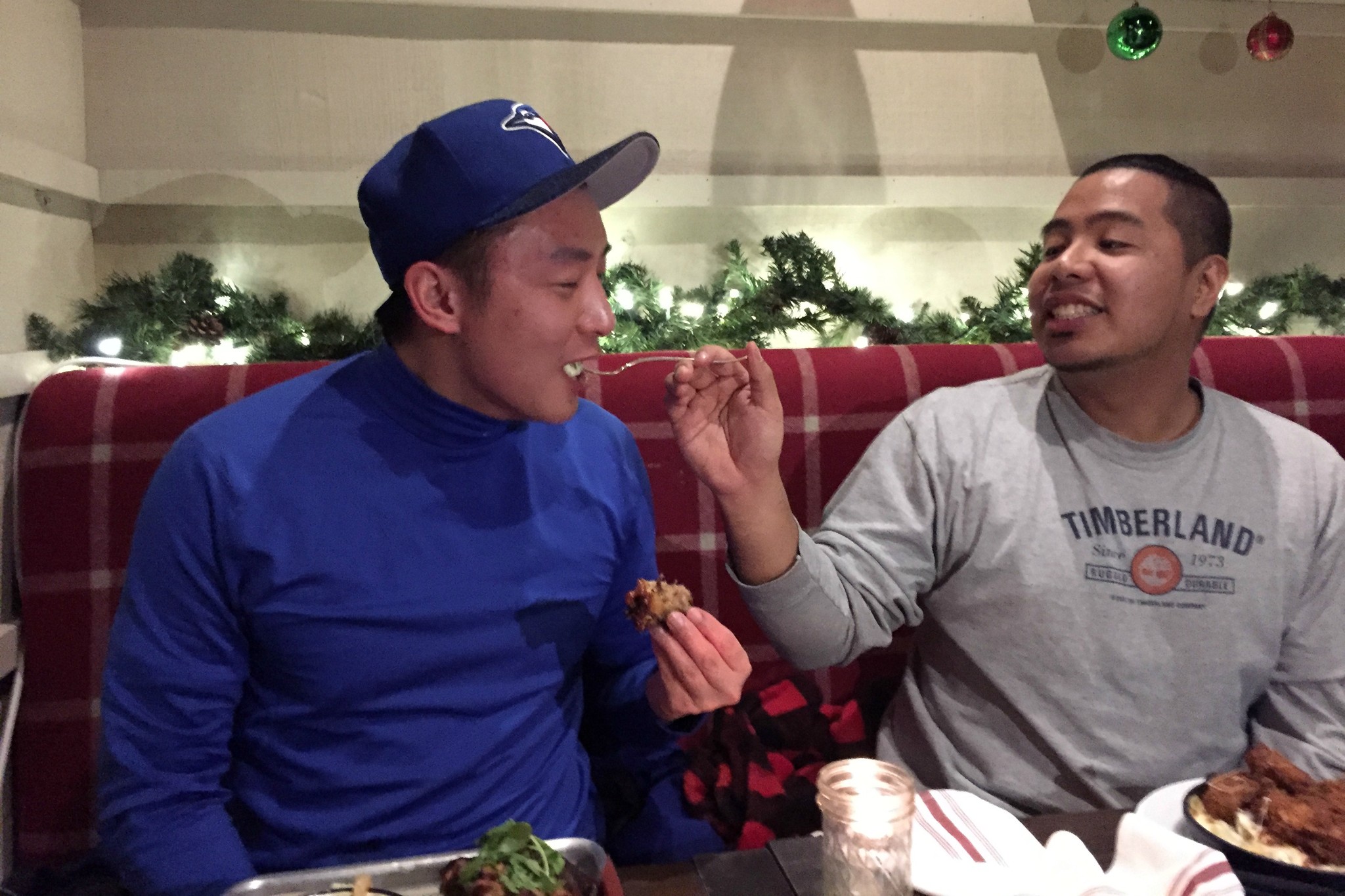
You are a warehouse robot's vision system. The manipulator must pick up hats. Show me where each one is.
[356,98,658,294]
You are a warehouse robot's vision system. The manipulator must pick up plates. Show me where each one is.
[1185,783,1345,876]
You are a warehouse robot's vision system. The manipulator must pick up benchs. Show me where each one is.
[0,335,1345,896]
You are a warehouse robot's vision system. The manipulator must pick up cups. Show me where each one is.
[816,756,916,895]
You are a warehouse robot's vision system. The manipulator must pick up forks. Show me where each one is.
[581,353,753,375]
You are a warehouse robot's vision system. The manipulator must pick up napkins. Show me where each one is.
[910,776,1245,896]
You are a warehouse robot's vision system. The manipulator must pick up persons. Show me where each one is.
[665,152,1345,819]
[98,97,751,896]
